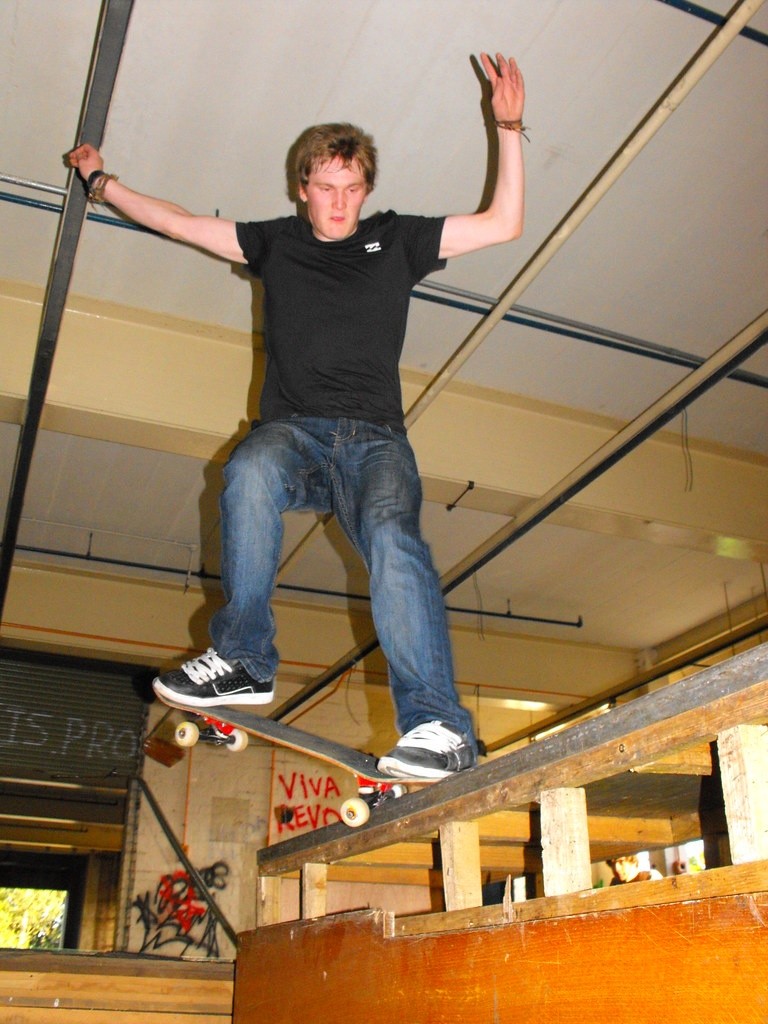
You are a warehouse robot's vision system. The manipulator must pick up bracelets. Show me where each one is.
[87,169,119,207]
[493,120,532,143]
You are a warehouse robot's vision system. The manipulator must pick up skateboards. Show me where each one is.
[149,676,447,832]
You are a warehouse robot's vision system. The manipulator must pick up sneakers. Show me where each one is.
[153,647,275,708]
[377,720,476,780]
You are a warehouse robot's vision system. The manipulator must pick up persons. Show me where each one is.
[69,52,524,778]
[604,856,665,886]
